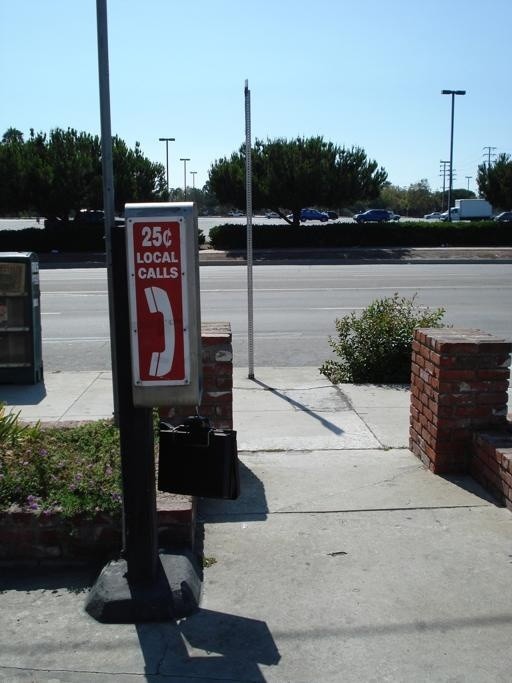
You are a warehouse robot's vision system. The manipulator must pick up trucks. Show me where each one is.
[439,198,492,222]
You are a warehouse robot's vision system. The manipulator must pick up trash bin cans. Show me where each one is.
[0,251,42,387]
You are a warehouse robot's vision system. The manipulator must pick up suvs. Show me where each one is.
[353,208,390,223]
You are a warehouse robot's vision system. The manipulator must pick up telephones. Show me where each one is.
[142,285,179,378]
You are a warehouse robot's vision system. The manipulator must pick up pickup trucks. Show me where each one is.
[43,208,125,229]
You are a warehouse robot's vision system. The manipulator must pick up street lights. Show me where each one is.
[440,88,466,222]
[190,170,197,188]
[158,137,176,193]
[180,158,190,194]
[464,175,473,191]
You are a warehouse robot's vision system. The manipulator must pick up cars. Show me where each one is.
[265,208,339,222]
[494,211,512,222]
[424,211,440,218]
[387,210,400,221]
[199,207,255,217]
